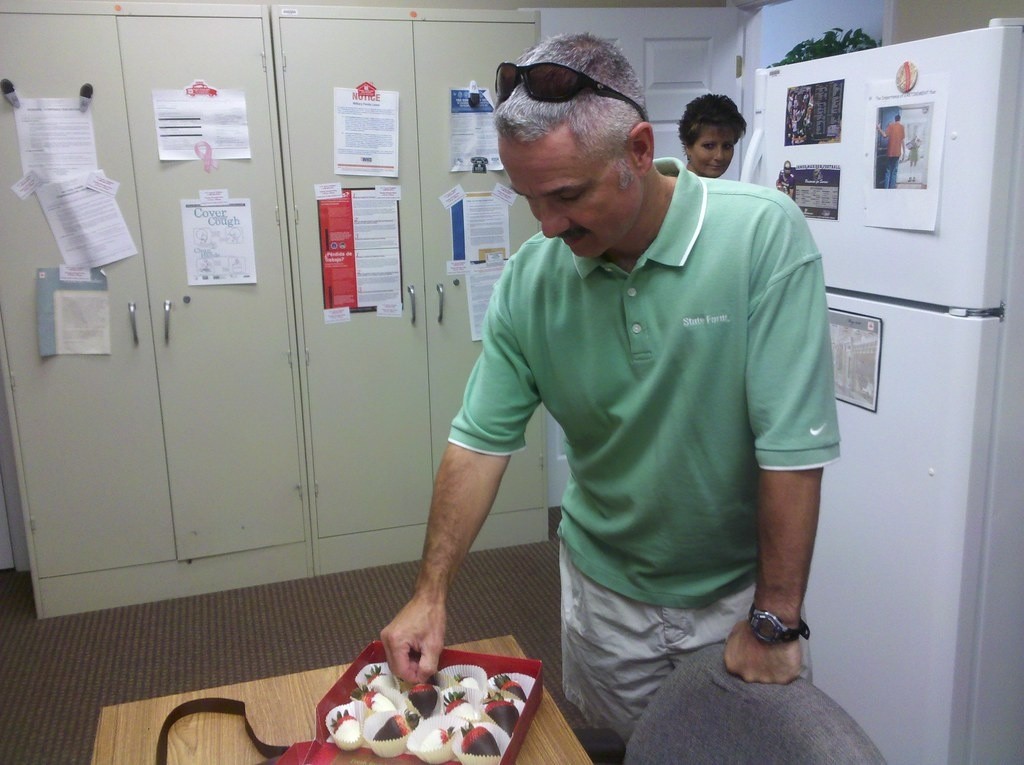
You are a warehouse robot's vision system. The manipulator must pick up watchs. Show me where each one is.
[747,600,810,646]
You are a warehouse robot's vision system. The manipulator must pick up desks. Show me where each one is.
[88,633,593,765]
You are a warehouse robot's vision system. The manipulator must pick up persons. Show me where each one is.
[877,115,905,189]
[379,32,841,746]
[905,132,922,182]
[678,93,747,179]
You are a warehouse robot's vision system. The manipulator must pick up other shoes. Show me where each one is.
[908,179,915,182]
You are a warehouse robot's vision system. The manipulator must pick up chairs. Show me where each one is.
[574,640,889,765]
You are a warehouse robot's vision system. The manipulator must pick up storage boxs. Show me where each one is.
[270,638,543,765]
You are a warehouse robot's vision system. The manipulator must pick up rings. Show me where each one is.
[383,650,393,657]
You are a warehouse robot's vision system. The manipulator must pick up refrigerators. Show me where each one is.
[742,19,1023,765]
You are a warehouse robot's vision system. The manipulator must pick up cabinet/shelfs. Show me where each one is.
[272,6,551,578]
[2,0,313,622]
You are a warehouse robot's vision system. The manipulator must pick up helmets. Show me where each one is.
[784,161,791,169]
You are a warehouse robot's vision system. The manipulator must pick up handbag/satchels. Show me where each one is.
[906,142,914,149]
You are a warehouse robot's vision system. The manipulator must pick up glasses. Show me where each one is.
[495,63,646,122]
